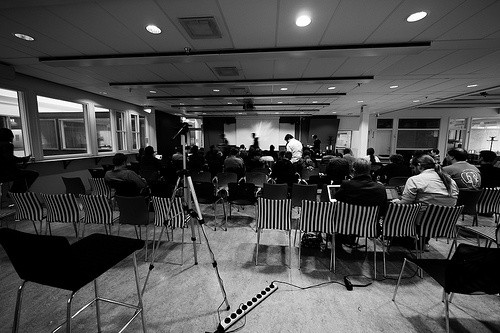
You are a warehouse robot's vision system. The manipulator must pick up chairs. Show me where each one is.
[392,243,500,333]
[0,191,202,266]
[0,226,147,333]
[256,187,500,279]
[61,163,408,231]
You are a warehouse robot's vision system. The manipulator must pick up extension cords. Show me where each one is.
[219,283,279,331]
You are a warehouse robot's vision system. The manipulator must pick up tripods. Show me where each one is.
[138,122,231,311]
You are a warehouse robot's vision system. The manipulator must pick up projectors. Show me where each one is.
[245,103,254,111]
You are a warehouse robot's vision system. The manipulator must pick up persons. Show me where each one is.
[440,150,481,189]
[104,153,151,198]
[267,143,500,213]
[127,133,264,198]
[0,127,38,193]
[333,158,388,246]
[312,135,321,157]
[285,134,303,178]
[389,154,459,251]
[476,150,500,189]
